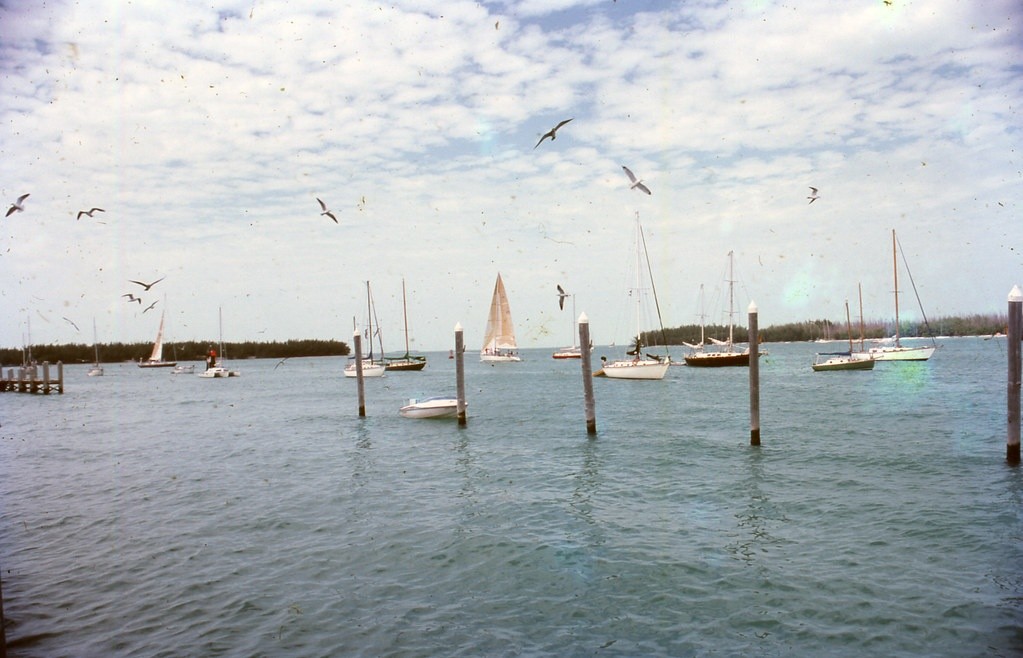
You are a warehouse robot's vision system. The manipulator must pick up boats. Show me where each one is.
[399,395,468,418]
[173,365,195,374]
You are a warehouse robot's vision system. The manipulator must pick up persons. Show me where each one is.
[206,353,212,371]
[211,349,216,367]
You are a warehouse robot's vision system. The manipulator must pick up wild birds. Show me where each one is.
[5,193,30,217]
[143,300,159,313]
[532,118,575,148]
[622,165,651,196]
[129,277,164,291]
[122,293,141,304]
[556,284,571,311]
[316,197,338,224]
[77,208,105,220]
[63,317,79,331]
[807,187,820,204]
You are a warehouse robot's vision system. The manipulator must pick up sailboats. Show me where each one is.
[20,312,35,374]
[553,294,581,357]
[479,272,520,362]
[198,308,239,378]
[602,211,671,380]
[343,277,427,377]
[88,317,102,377]
[813,299,875,372]
[138,294,177,368]
[869,229,936,361]
[684,250,767,366]
